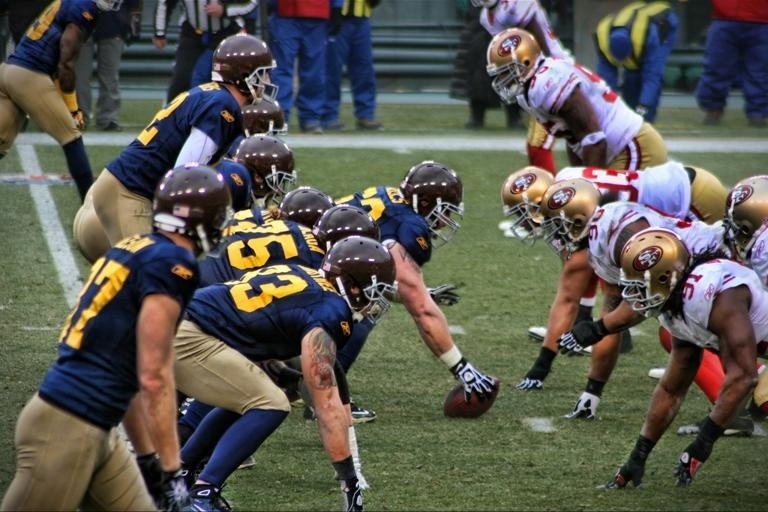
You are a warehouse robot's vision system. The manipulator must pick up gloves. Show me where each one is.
[606,432,657,491]
[556,319,609,358]
[333,455,364,512]
[515,345,557,392]
[135,451,194,512]
[563,377,604,421]
[427,282,461,306]
[438,344,499,404]
[673,414,726,488]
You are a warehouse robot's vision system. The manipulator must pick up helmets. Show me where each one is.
[240,96,288,136]
[150,163,231,242]
[280,186,397,329]
[212,33,278,94]
[541,178,600,243]
[485,26,543,106]
[235,133,296,204]
[723,174,767,262]
[399,159,466,249]
[500,164,555,228]
[616,227,693,314]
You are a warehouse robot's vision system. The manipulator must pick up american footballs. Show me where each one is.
[444,379,499,417]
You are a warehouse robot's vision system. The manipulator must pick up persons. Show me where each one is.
[592,1,683,124]
[694,1,768,129]
[450,0,542,129]
[72,31,499,511]
[2,162,230,510]
[499,161,768,488]
[154,0,379,129]
[486,29,671,356]
[0,0,145,210]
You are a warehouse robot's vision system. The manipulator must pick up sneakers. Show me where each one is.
[357,119,386,132]
[750,117,768,129]
[583,329,635,354]
[508,119,529,132]
[525,321,552,341]
[303,402,377,428]
[701,110,724,129]
[299,117,345,134]
[183,490,230,511]
[465,116,487,130]
[97,120,124,133]
